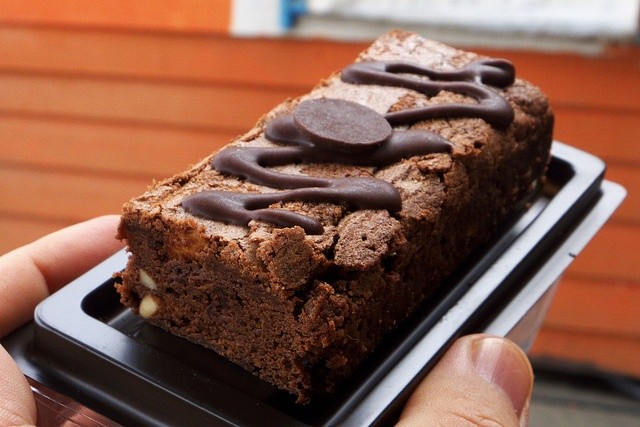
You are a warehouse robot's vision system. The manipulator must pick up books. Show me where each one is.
[281,0,640,41]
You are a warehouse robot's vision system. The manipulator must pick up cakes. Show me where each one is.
[120,29,556,400]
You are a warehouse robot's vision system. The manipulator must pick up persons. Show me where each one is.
[1,214,534,426]
